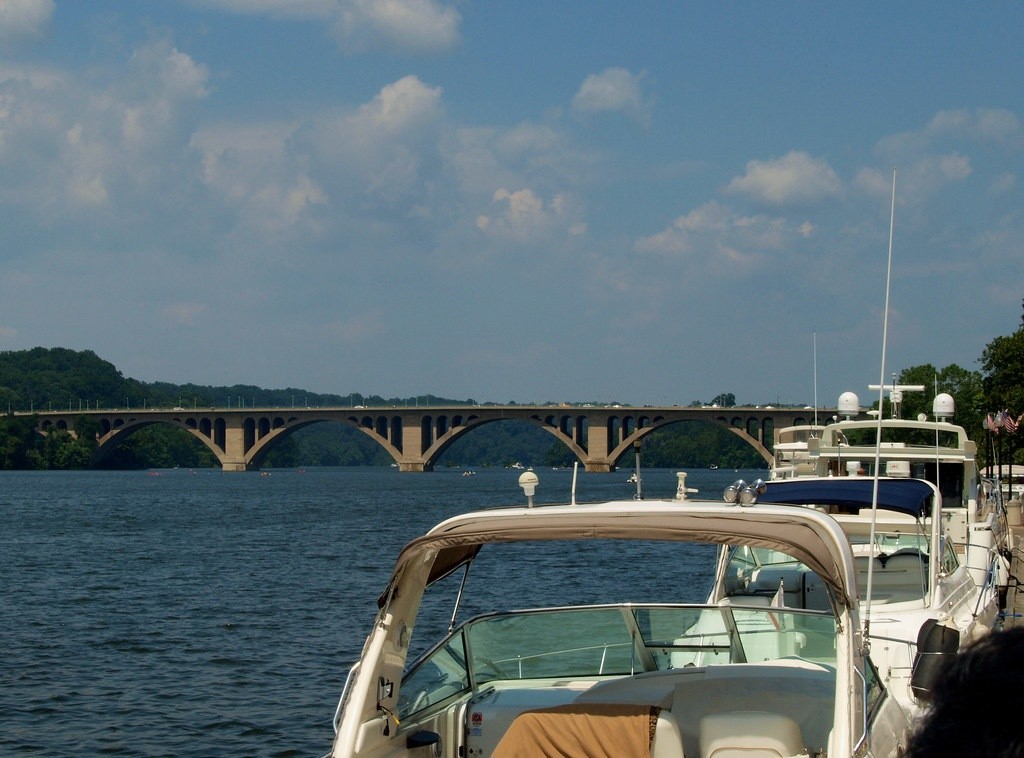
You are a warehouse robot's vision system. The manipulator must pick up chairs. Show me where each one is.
[490,703,803,758]
[713,553,928,665]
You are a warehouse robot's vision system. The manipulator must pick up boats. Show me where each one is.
[660,327,1024,733]
[305,166,912,758]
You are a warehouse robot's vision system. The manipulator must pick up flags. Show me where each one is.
[987,408,1024,435]
[766,581,785,630]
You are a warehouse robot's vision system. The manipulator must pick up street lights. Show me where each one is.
[994,410,1008,509]
[980,412,993,500]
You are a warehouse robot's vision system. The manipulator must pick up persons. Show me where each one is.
[905,624,1024,758]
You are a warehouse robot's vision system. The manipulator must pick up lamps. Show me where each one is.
[723,479,768,507]
